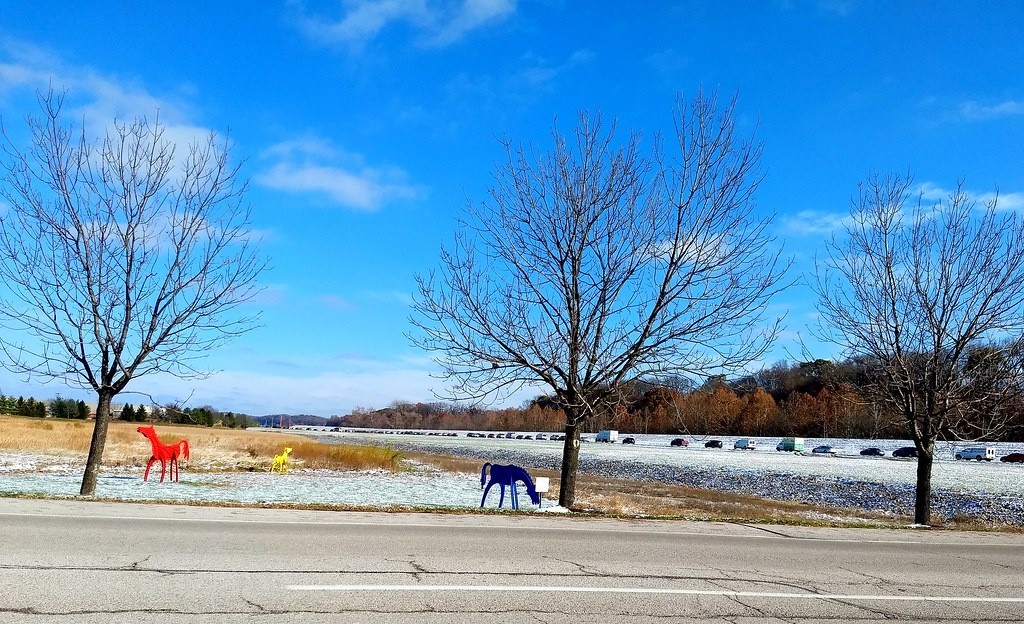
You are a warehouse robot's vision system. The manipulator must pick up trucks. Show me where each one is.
[776,437,804,452]
[595,430,618,443]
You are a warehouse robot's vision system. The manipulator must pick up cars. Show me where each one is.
[812,445,837,455]
[1000,453,1024,463]
[467,432,595,442]
[860,447,885,457]
[892,447,919,458]
[705,440,723,449]
[623,438,635,444]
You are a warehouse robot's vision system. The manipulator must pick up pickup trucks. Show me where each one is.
[671,438,688,447]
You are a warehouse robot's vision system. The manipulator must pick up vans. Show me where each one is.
[734,439,756,451]
[955,447,995,462]
